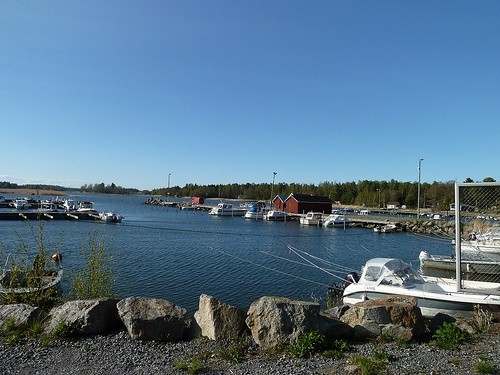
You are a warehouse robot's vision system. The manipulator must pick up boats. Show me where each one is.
[98,210,123,223]
[322,214,350,228]
[451,232,500,255]
[299,212,327,225]
[144,197,191,208]
[418,251,500,275]
[0,194,97,212]
[332,206,371,216]
[243,207,279,219]
[262,210,292,221]
[341,256,500,322]
[207,200,250,216]
[373,221,407,233]
[432,214,447,221]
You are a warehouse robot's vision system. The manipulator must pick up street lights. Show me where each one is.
[270,172,277,207]
[418,158,424,216]
